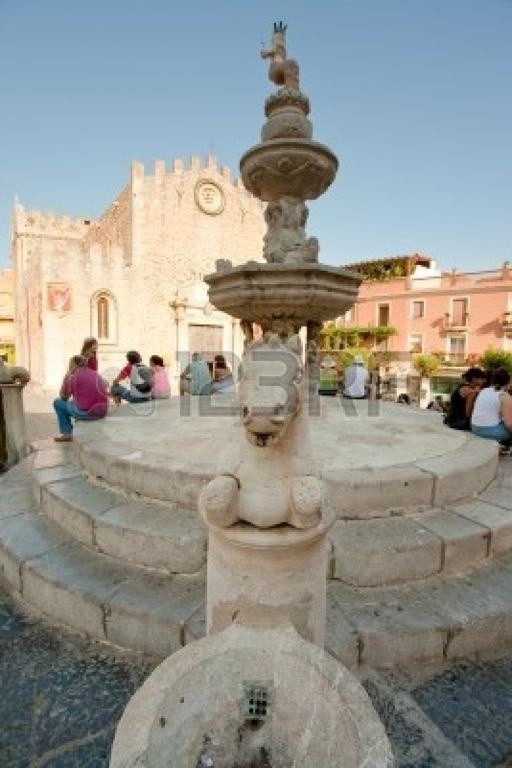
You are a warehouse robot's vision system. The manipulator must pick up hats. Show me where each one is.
[319,354,337,368]
[353,355,364,364]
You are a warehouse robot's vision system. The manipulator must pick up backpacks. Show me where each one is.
[135,368,154,392]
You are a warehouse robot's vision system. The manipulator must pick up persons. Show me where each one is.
[469,367,511,460]
[426,394,451,413]
[342,353,372,399]
[0,386,11,472]
[442,366,488,432]
[50,353,111,441]
[395,391,411,405]
[211,352,233,392]
[147,353,171,399]
[106,348,157,408]
[76,334,99,373]
[176,349,215,397]
[316,355,339,395]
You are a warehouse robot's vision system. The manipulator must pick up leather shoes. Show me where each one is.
[53,435,71,442]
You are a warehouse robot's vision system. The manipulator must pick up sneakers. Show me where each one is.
[501,446,511,455]
